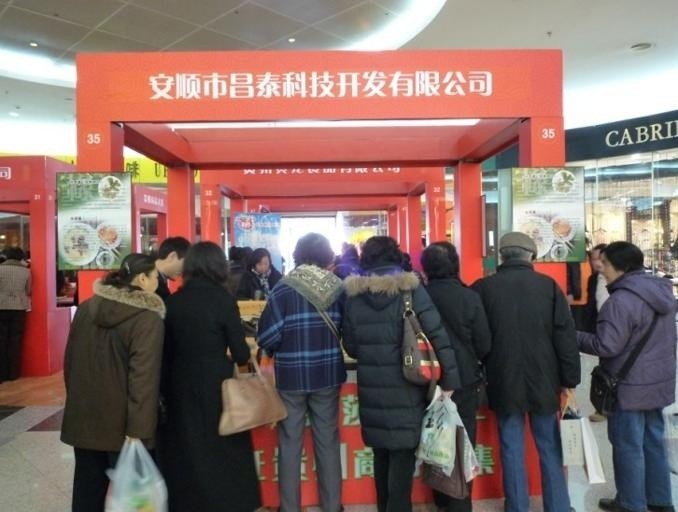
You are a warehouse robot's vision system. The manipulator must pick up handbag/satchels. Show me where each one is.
[340,339,356,365]
[215,373,289,438]
[404,313,444,384]
[592,367,618,417]
[425,425,469,500]
[558,405,604,483]
[257,349,276,430]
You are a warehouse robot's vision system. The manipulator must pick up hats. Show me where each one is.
[500,233,538,259]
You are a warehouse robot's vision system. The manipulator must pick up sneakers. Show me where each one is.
[590,412,607,423]
[597,497,630,512]
[648,505,676,511]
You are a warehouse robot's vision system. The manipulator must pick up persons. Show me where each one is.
[1,243,32,384]
[467,231,580,512]
[340,234,461,512]
[573,240,677,511]
[135,234,676,427]
[253,234,347,511]
[162,241,263,511]
[56,253,168,512]
